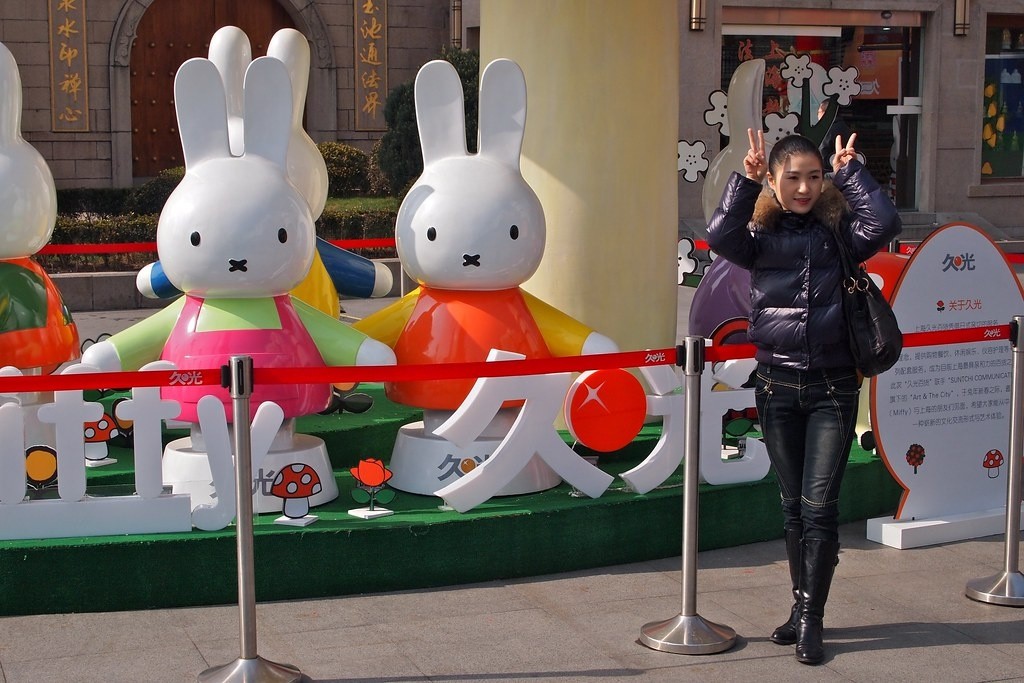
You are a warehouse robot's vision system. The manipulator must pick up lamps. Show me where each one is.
[881,11,892,18]
[690,0,707,31]
[954,0,970,36]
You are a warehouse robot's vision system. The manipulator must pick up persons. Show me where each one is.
[705,128,902,663]
[818,98,830,121]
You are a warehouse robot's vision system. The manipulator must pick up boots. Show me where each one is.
[770,527,802,645]
[795,538,841,663]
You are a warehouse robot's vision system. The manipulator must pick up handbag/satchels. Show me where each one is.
[830,216,903,378]
